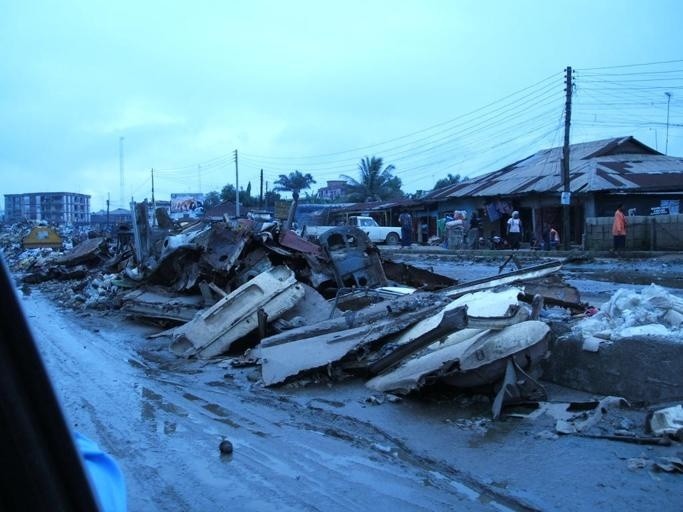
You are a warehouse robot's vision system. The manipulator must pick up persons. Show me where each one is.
[549,224,560,246]
[506,211,522,250]
[612,202,628,250]
[469,212,479,250]
[421,219,429,242]
[398,208,412,247]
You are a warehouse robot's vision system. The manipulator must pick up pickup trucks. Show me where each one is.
[305,217,402,245]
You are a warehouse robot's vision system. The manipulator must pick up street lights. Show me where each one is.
[664,93,671,154]
